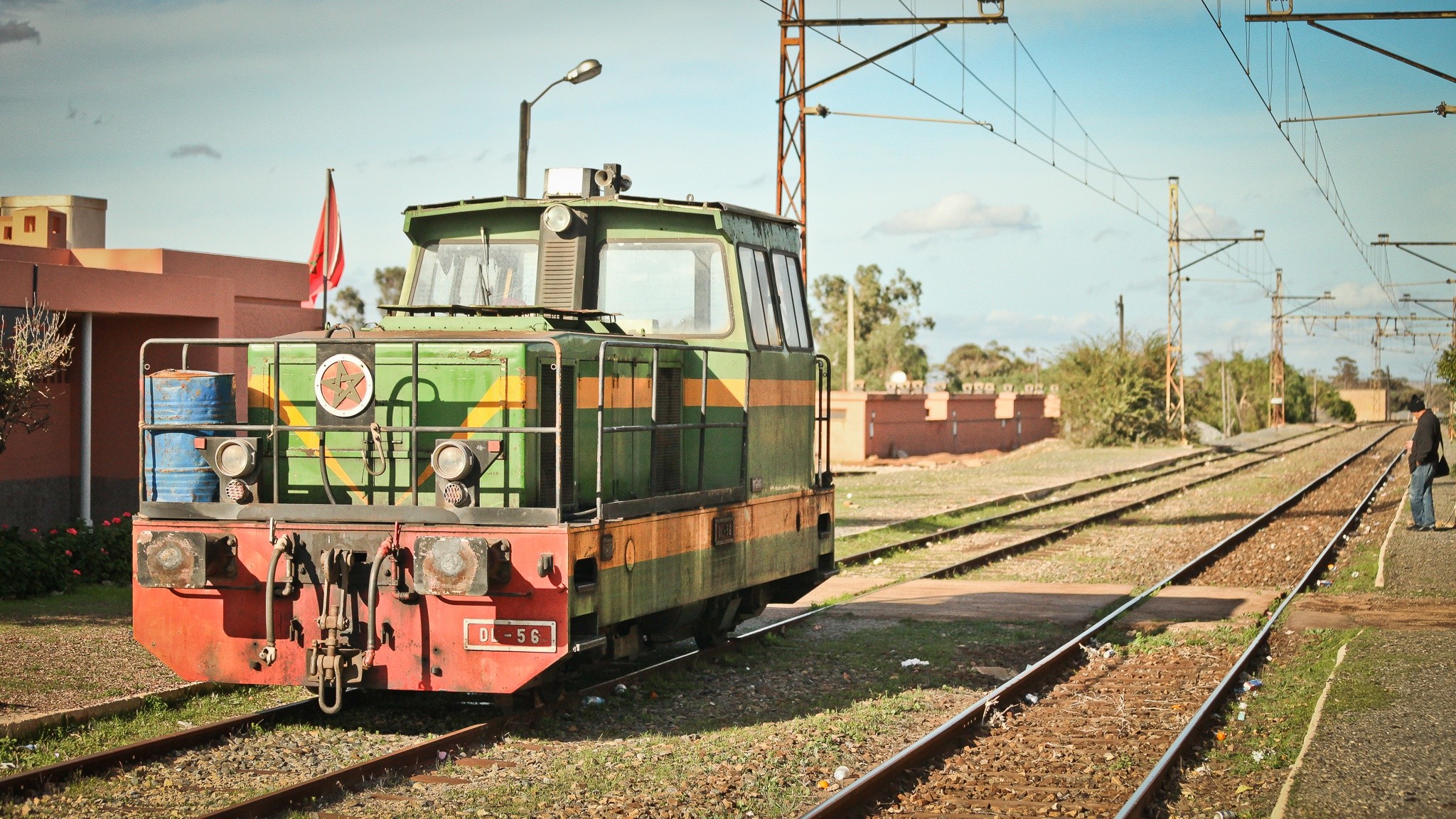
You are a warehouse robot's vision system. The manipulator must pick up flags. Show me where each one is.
[300,171,345,311]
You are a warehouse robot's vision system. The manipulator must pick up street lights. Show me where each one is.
[516,59,603,198]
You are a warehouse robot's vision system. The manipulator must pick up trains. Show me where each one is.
[131,165,836,711]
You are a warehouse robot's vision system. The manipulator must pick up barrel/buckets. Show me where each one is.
[143,369,237,502]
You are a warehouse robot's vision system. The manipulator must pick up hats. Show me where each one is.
[1408,394,1426,411]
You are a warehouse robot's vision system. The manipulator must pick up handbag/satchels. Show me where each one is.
[1434,455,1450,477]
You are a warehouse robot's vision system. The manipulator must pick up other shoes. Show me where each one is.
[1406,524,1422,531]
[1418,527,1436,532]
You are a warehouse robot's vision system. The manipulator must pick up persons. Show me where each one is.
[1404,395,1441,532]
